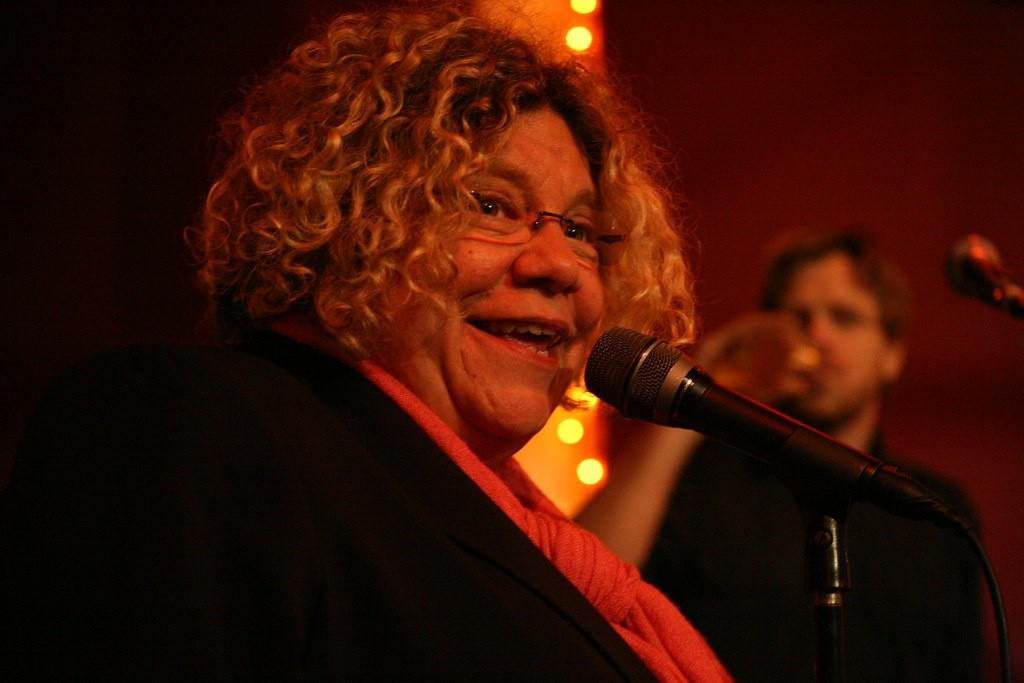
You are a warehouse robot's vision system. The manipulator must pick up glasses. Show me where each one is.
[457,185,631,265]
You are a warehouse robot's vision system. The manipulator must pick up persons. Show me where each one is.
[0,0,732,683]
[573,221,983,681]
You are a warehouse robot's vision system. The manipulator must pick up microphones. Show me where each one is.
[943,233,1024,318]
[584,325,929,505]
